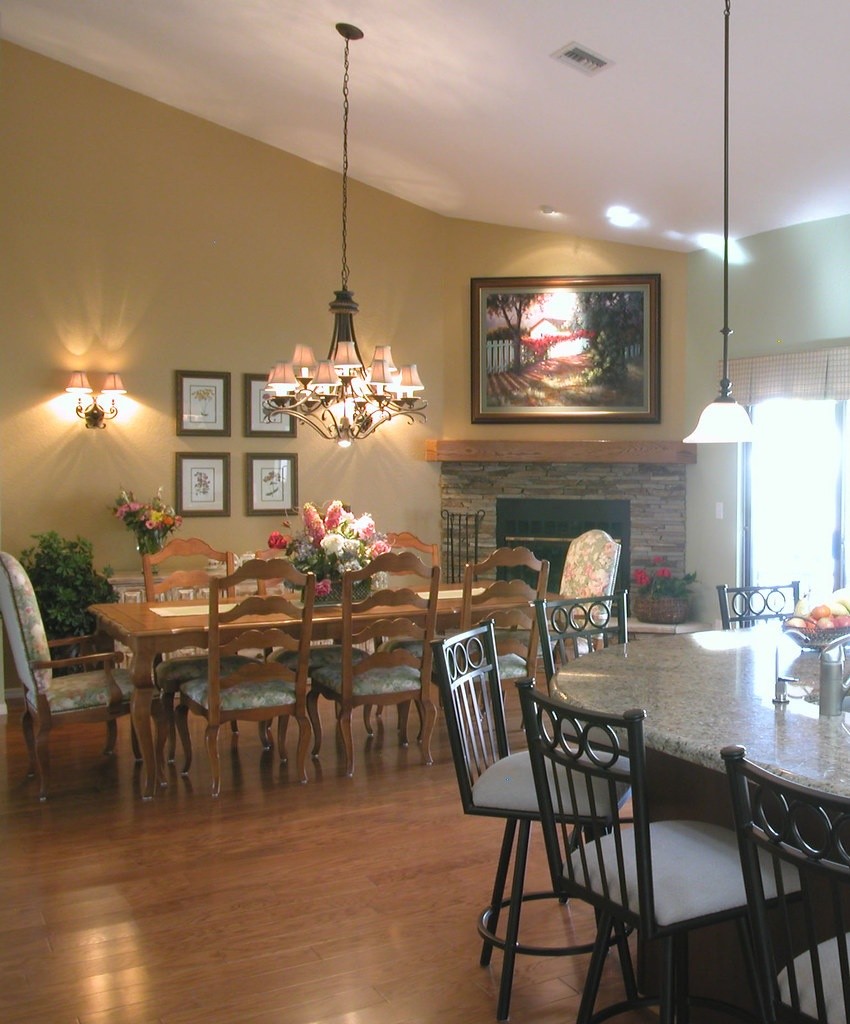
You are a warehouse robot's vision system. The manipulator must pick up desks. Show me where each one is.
[548,625,849,1024]
[87,579,568,800]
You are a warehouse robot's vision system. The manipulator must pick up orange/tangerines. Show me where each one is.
[805,605,830,632]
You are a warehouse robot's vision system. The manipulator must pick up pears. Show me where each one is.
[822,589,850,616]
[787,595,808,632]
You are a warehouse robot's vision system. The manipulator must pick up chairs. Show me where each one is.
[720,745,849,1024]
[514,677,804,1024]
[534,590,630,757]
[429,618,634,1024]
[717,581,800,630]
[0,529,623,802]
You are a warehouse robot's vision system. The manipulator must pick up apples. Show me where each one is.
[815,615,850,630]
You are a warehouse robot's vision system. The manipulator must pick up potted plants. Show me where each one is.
[16,530,122,679]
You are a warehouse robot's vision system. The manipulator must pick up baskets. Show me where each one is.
[634,579,691,624]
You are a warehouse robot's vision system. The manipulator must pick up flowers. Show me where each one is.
[633,557,701,600]
[107,483,183,555]
[269,499,392,592]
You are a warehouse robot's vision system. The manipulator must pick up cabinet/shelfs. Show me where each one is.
[111,570,289,668]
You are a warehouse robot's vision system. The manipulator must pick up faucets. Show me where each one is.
[815,634,850,718]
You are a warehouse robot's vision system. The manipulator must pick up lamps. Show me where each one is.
[66,372,126,430]
[683,1,755,444]
[262,22,426,445]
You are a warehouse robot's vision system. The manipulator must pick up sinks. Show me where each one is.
[816,696,850,713]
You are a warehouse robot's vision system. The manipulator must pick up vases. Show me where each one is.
[302,578,372,604]
[135,531,167,574]
[635,593,688,624]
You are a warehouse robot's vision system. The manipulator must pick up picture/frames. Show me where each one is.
[175,452,232,517]
[470,273,661,425]
[244,373,297,438]
[246,452,298,517]
[175,370,232,437]
